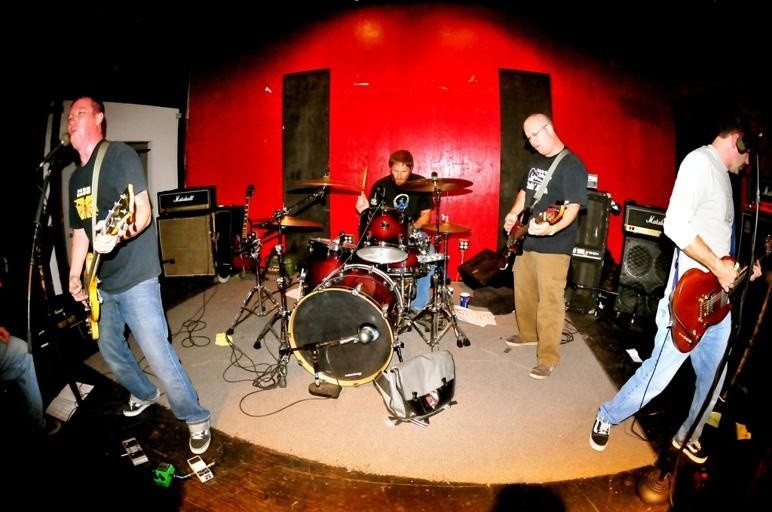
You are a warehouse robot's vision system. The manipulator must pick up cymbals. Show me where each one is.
[399,178,473,192]
[290,181,358,188]
[421,224,470,233]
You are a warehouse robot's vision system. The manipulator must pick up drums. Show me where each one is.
[288,264,403,385]
[357,206,408,264]
[387,247,420,274]
[306,238,355,293]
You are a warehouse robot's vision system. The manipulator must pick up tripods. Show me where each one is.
[394,232,429,346]
[228,243,282,336]
[401,190,472,350]
[255,192,323,358]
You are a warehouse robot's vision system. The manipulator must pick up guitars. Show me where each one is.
[670,235,772,353]
[82,184,135,340]
[503,203,566,256]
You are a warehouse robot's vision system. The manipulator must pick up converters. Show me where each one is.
[152,462,175,488]
[123,437,148,467]
[186,455,214,483]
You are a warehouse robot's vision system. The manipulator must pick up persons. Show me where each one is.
[59,95,214,455]
[590,124,762,466]
[367,148,438,315]
[0,326,65,449]
[503,111,589,381]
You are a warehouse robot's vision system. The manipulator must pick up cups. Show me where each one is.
[459,292,470,308]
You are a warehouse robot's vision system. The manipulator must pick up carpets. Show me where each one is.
[78,280,660,489]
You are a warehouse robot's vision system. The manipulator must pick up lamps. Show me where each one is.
[353,72,370,86]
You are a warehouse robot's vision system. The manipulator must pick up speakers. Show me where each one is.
[564,257,604,315]
[614,234,674,321]
[158,206,244,280]
[563,190,611,260]
[456,246,505,290]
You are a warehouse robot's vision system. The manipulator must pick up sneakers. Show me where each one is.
[506,335,540,347]
[673,436,709,463]
[124,389,160,417]
[530,364,556,379]
[589,406,612,450]
[189,421,211,454]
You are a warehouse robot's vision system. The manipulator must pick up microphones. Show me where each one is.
[736,124,772,153]
[431,172,438,187]
[40,132,71,168]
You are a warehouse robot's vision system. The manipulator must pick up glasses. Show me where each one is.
[527,123,548,139]
